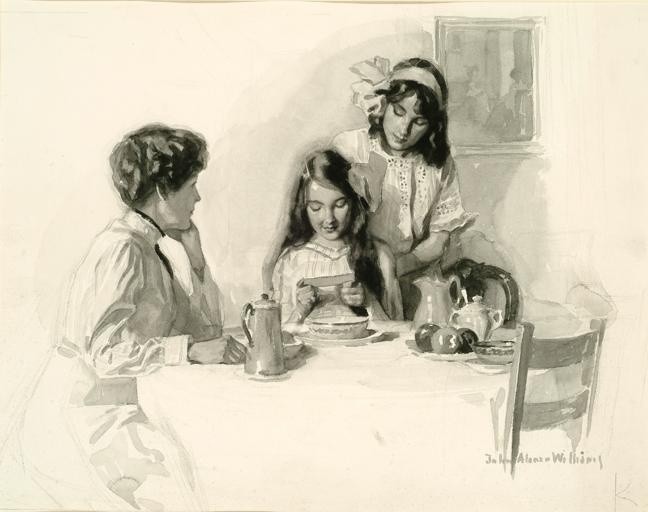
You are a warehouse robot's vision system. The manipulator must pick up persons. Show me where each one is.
[257,146,408,327]
[18,124,249,510]
[330,54,480,323]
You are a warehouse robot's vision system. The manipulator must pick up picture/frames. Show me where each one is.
[432,16,542,157]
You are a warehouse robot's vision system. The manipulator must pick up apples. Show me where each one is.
[415,323,439,353]
[431,326,460,354]
[456,328,479,354]
